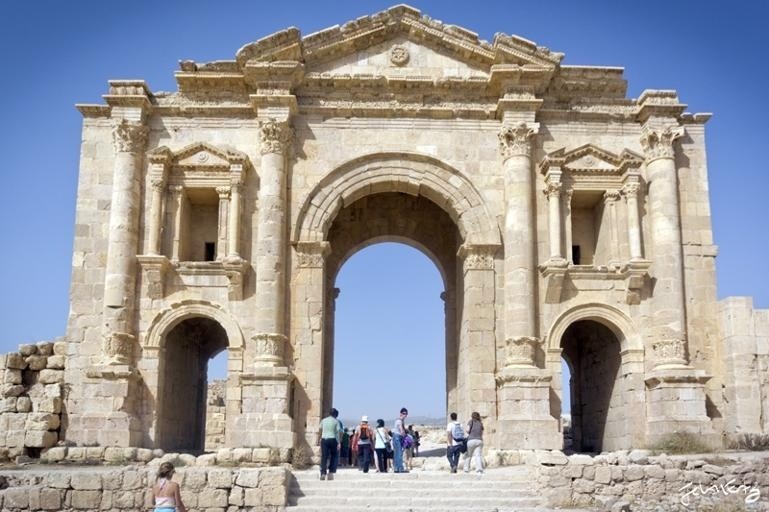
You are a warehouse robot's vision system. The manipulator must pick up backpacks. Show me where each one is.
[358,424,370,440]
[452,422,465,442]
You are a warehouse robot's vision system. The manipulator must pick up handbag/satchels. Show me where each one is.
[463,420,473,451]
[384,442,394,458]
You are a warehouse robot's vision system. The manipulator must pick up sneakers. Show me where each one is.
[319,472,334,481]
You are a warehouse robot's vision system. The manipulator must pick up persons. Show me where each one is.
[372,418,391,473]
[447,412,462,473]
[314,407,341,480]
[350,414,372,473]
[464,412,483,473]
[151,461,187,512]
[338,426,421,469]
[392,407,410,473]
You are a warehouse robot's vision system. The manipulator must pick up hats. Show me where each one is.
[360,416,368,422]
